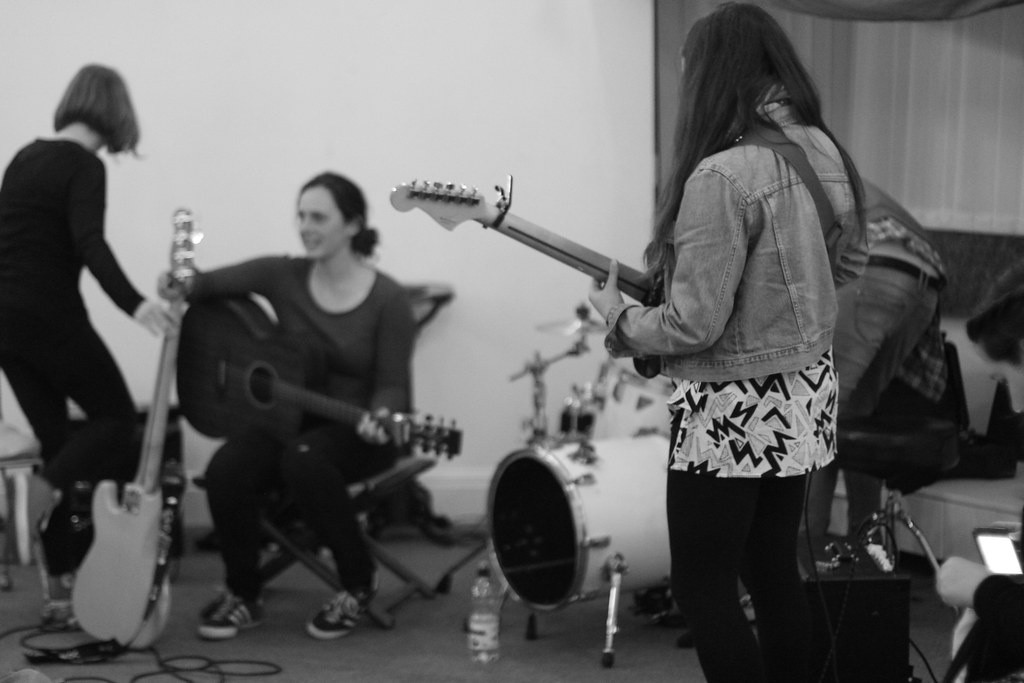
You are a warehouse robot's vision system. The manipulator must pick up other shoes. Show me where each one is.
[738,595,756,623]
[40,573,76,622]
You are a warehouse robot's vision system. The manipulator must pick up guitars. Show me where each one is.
[388,173,647,306]
[71,208,206,650]
[176,295,465,460]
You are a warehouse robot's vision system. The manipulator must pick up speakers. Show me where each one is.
[794,530,910,683]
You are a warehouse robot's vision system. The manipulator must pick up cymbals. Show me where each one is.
[537,316,608,336]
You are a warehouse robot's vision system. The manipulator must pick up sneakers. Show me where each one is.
[306,578,378,640]
[197,595,263,639]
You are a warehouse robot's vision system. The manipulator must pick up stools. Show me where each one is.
[193,458,435,628]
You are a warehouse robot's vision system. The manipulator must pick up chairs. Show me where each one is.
[823,316,969,616]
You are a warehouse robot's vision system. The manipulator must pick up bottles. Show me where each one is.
[466,569,501,665]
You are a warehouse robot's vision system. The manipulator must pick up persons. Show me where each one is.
[589,0,1024,682]
[156,172,414,639]
[0,65,182,593]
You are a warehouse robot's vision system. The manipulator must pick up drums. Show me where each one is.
[485,434,671,613]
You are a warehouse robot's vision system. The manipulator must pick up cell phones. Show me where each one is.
[972,526,1023,575]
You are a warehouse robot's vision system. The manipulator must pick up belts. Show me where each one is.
[866,255,942,291]
